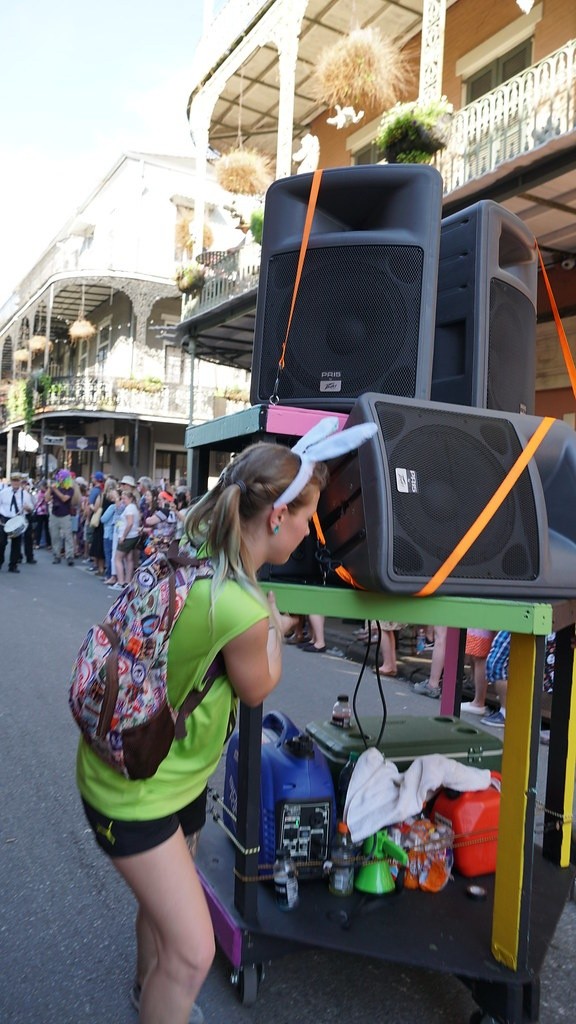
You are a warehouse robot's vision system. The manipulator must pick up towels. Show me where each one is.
[342,745,491,844]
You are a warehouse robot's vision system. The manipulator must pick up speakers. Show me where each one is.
[250,165,575,601]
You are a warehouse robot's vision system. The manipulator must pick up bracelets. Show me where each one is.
[269,626,276,630]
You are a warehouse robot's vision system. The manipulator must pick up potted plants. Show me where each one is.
[24,374,50,426]
[209,387,249,419]
[376,111,446,164]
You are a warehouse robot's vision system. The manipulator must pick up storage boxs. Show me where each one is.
[306,715,506,811]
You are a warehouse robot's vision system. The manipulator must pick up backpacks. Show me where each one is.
[70,538,251,781]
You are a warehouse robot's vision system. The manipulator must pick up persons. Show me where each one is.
[327,109,363,129]
[460,628,556,743]
[352,619,448,698]
[0,469,190,590]
[284,614,327,653]
[74,443,323,1024]
[293,133,320,176]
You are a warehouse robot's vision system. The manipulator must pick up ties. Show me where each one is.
[9,489,20,515]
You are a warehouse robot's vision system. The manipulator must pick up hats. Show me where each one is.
[11,472,21,481]
[91,472,104,480]
[21,473,29,482]
[117,475,137,489]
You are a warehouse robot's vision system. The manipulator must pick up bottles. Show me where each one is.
[416,628,427,657]
[272,849,300,911]
[329,821,355,898]
[330,694,352,729]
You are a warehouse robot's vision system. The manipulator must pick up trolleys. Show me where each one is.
[191,582,576,1024]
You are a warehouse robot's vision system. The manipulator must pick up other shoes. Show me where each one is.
[31,541,129,592]
[352,626,369,634]
[26,558,36,564]
[460,701,487,715]
[298,641,327,654]
[363,636,380,644]
[130,981,205,1024]
[480,710,506,728]
[357,632,372,640]
[286,633,306,645]
[68,557,74,565]
[8,566,20,574]
[373,666,397,678]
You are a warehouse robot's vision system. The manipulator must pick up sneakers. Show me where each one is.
[413,680,441,698]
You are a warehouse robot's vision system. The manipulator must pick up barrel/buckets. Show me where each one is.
[222,709,338,883]
[429,770,502,877]
